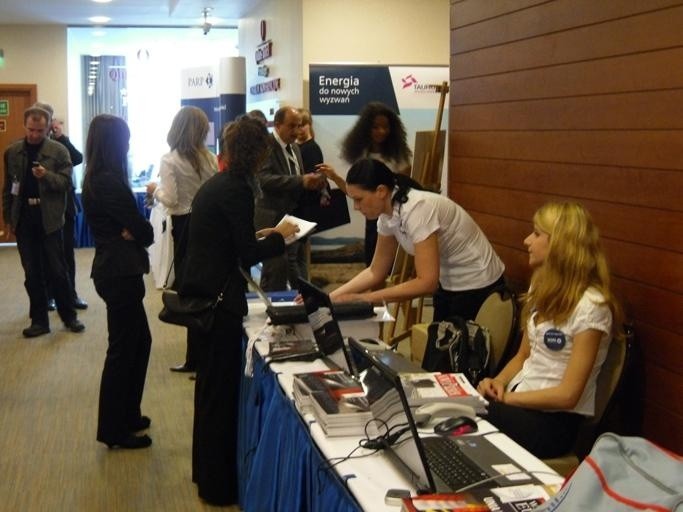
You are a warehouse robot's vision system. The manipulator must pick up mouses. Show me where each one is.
[434,416,478,436]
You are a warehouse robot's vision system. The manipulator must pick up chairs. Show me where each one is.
[473,285,521,378]
[541,323,638,475]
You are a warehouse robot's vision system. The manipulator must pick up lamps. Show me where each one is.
[203,9,211,35]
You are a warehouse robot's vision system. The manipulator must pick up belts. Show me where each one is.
[23,198,40,205]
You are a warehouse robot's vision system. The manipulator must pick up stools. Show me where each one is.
[412,324,429,368]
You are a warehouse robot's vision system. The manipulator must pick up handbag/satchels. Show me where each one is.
[159,290,213,325]
[525,432,683,512]
[299,181,350,233]
[423,318,492,387]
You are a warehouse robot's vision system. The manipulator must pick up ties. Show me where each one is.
[286,144,296,175]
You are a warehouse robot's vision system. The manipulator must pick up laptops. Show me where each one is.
[348,336,545,493]
[236,257,305,309]
[297,275,427,374]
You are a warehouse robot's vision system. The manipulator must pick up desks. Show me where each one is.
[72,187,157,249]
[236,290,568,512]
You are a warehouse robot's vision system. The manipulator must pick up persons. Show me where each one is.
[80,114,154,450]
[1,102,88,339]
[293,157,507,374]
[147,105,219,381]
[247,105,413,292]
[180,114,300,508]
[476,199,630,460]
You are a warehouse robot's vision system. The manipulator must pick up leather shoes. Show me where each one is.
[23,296,87,336]
[169,364,196,372]
[107,417,151,449]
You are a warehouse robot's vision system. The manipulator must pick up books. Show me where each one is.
[402,484,551,512]
[291,366,491,439]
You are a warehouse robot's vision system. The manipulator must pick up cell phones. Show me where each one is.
[33,162,39,167]
[52,127,56,133]
